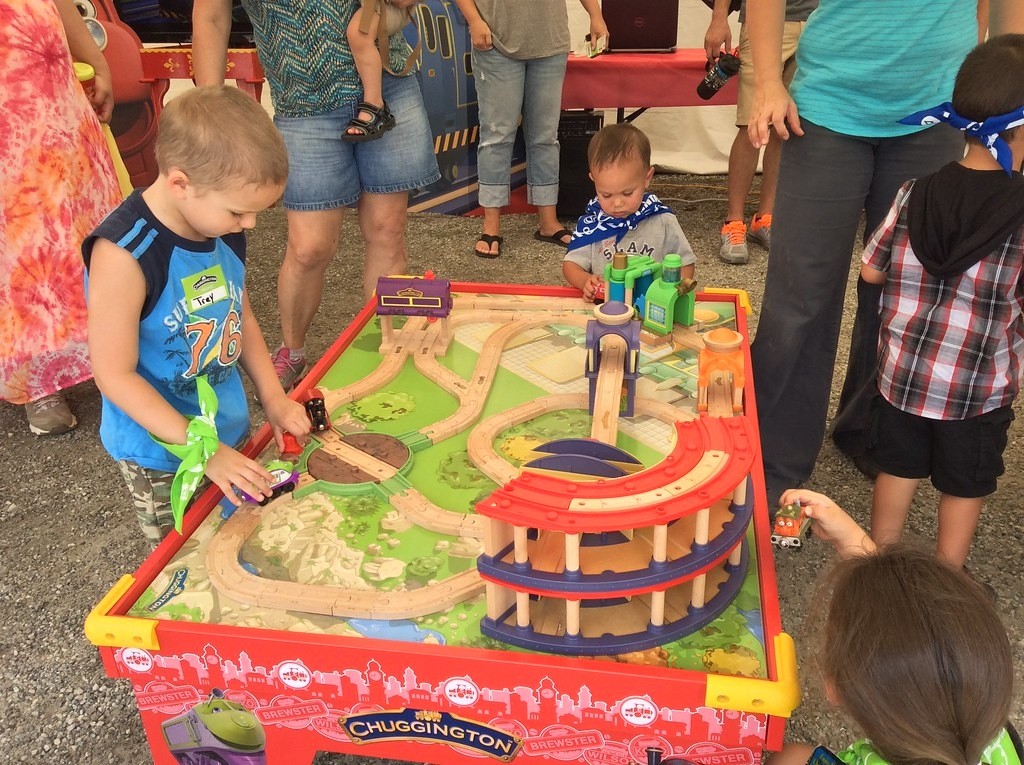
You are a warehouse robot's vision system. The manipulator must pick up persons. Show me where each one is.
[0,0,125,435]
[736,0,1024,520]
[765,489,1024,765]
[861,33,1024,570]
[563,124,697,303]
[456,0,609,262]
[704,0,819,264]
[81,86,311,552]
[192,0,441,404]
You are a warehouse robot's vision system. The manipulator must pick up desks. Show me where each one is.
[559,48,740,128]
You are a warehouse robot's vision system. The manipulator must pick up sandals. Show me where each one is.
[341,100,397,142]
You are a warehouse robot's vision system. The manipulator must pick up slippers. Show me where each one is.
[533,226,572,248]
[476,232,503,260]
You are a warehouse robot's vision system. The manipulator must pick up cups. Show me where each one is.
[697,51,741,100]
[71,62,95,111]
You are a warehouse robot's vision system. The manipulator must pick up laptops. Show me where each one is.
[601,0,679,53]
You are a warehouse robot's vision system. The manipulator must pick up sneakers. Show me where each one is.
[251,344,309,406]
[720,220,749,263]
[746,213,776,251]
[24,391,76,437]
